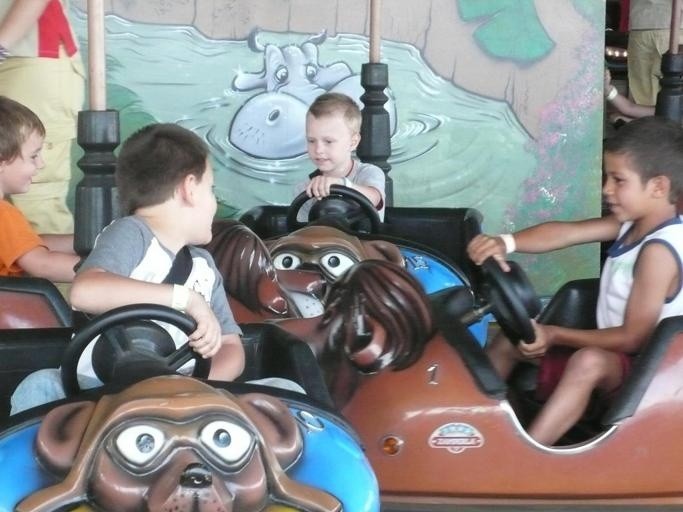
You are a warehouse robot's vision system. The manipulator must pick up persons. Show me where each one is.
[466,115,683,446]
[9,122,307,415]
[292,92,386,223]
[0,95,82,284]
